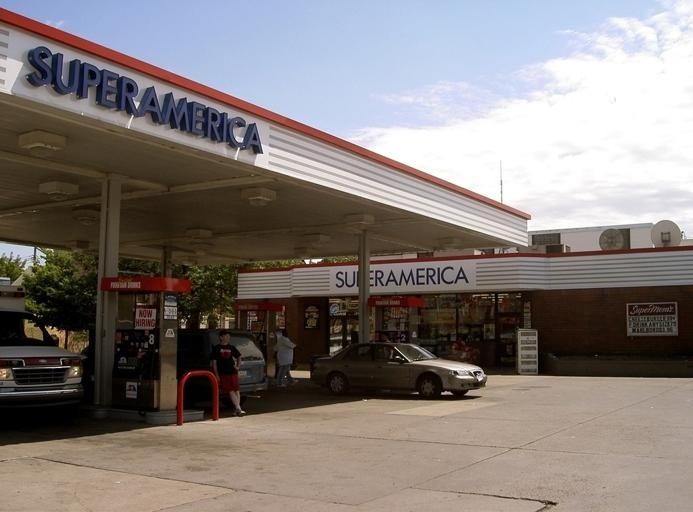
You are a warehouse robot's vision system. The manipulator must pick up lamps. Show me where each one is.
[65,240,89,250]
[178,256,196,265]
[192,243,210,256]
[73,208,100,225]
[440,237,469,248]
[39,181,79,201]
[18,130,68,154]
[185,229,214,241]
[344,214,375,226]
[240,187,277,207]
[304,234,331,242]
[294,247,321,257]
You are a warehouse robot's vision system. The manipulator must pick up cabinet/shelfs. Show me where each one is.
[516,329,540,376]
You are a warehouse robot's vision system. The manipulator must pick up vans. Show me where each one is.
[80,330,268,404]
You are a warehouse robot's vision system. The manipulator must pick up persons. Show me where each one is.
[212,330,246,417]
[272,329,298,387]
[380,332,394,359]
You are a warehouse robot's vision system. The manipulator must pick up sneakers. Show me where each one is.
[276,377,299,388]
[232,408,246,417]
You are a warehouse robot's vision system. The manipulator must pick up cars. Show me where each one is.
[0,308,86,407]
[311,342,487,398]
